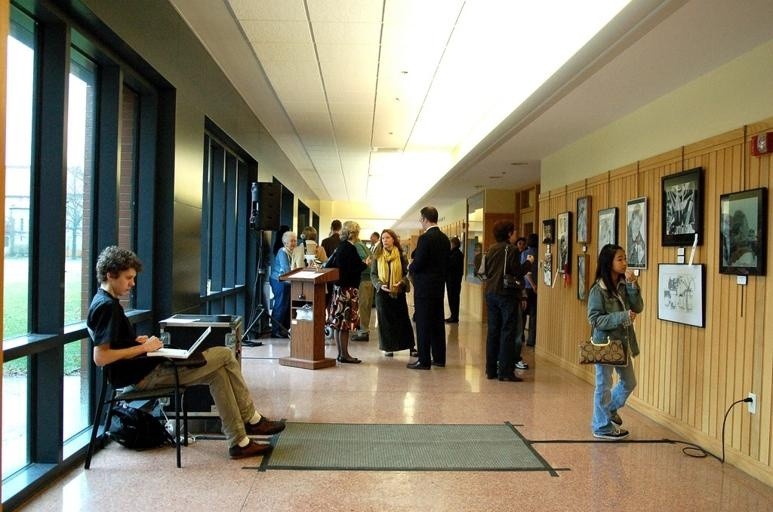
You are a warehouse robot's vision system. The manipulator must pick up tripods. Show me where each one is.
[241,230,290,342]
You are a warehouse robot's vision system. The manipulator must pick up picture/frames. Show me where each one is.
[557,211,573,273]
[577,254,590,301]
[576,195,592,244]
[626,196,648,270]
[542,218,555,244]
[658,263,705,328]
[661,167,704,247]
[598,206,619,261]
[719,186,768,276]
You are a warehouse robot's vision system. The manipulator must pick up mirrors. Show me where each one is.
[466,189,484,284]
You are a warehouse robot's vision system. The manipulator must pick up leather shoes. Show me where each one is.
[385,352,393,356]
[337,355,361,363]
[407,349,445,369]
[446,316,459,322]
[351,334,369,341]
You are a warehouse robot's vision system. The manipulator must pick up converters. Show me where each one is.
[175,436,193,444]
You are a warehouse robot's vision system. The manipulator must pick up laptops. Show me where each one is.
[147,326,211,359]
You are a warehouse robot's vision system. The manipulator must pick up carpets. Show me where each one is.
[259,418,549,472]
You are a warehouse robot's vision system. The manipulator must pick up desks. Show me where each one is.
[160,313,242,441]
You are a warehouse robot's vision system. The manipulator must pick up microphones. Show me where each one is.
[301,234,306,243]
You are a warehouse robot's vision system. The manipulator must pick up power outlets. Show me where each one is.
[747,393,756,414]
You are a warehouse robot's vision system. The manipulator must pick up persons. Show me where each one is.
[587,244,643,441]
[485,221,538,382]
[474,243,482,278]
[87,245,285,459]
[627,205,645,265]
[729,211,756,268]
[268,204,464,369]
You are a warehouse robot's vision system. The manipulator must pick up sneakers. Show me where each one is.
[610,410,623,425]
[593,425,629,439]
[515,359,528,369]
[246,413,285,434]
[228,438,271,458]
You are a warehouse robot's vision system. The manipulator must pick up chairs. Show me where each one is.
[85,356,187,470]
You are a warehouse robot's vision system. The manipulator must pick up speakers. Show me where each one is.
[249,182,282,230]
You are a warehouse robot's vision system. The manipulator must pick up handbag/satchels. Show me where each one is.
[504,273,524,288]
[107,404,168,450]
[579,335,628,366]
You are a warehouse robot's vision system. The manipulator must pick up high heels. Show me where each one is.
[498,371,521,382]
[485,368,497,379]
